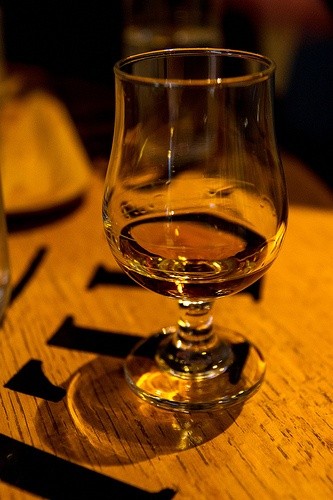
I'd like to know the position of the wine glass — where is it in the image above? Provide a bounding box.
[100,42,291,413]
